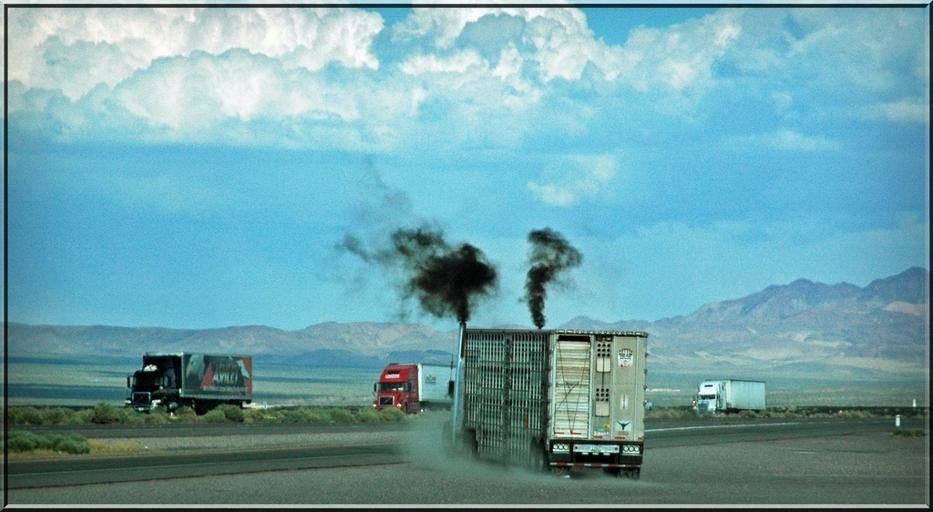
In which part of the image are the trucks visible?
[442,321,648,481]
[691,380,766,417]
[125,352,252,415]
[373,361,456,414]
